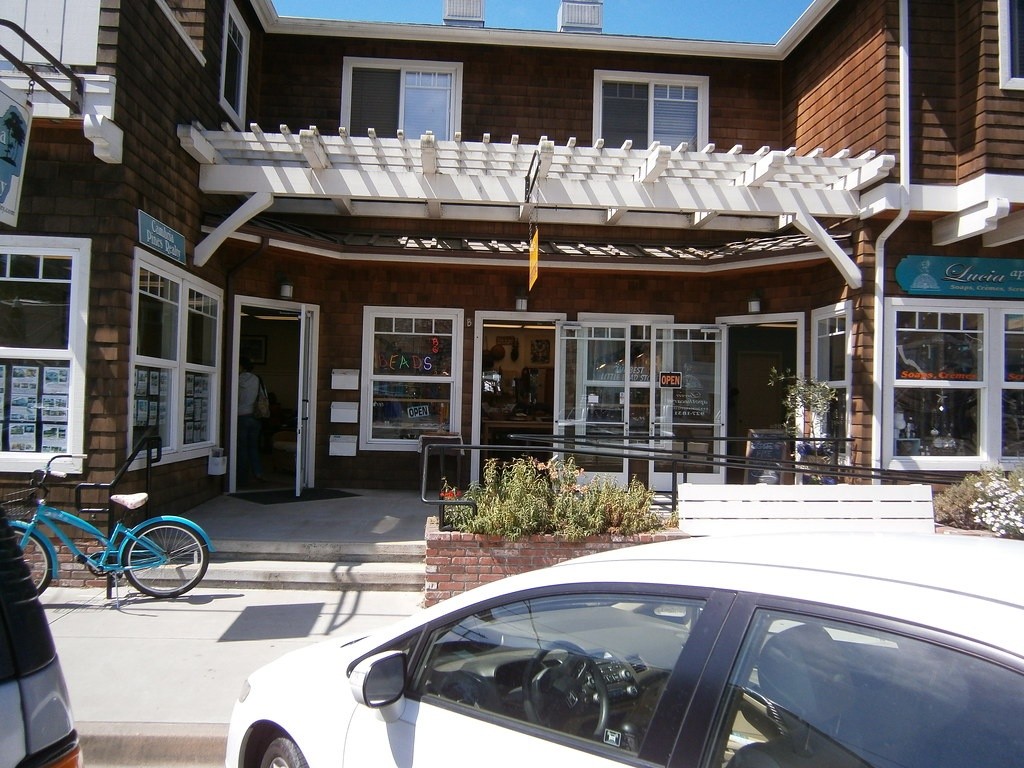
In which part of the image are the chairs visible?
[727,624,863,768]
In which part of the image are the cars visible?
[224,522,1024,768]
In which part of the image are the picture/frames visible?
[240,335,268,365]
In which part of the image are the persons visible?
[482,349,500,403]
[237,356,270,487]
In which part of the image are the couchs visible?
[271,430,297,473]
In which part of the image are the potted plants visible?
[426,453,691,611]
[767,367,842,474]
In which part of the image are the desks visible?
[482,403,553,479]
[418,435,463,494]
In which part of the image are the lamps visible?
[745,296,761,313]
[515,290,528,312]
[595,344,644,372]
[274,272,296,300]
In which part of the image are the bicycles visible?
[0,453,216,609]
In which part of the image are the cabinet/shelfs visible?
[372,398,450,431]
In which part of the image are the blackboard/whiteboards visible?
[672,388,711,419]
[742,429,789,485]
[592,362,650,394]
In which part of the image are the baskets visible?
[0,488,37,520]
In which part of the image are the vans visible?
[0,504,86,768]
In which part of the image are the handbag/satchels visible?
[256,374,270,418]
